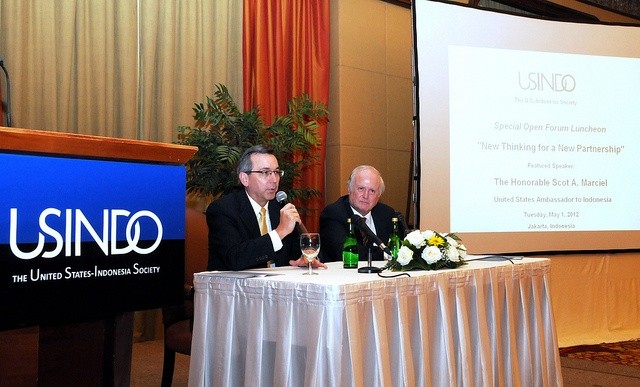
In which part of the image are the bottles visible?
[387,217,401,265]
[342,217,359,268]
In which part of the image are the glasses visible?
[244,170,284,178]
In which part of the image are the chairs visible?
[160,208,210,386]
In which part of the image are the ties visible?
[259,208,270,268]
[362,218,368,247]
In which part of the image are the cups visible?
[300,233,320,277]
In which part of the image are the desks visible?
[187,253,565,387]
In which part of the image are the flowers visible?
[395,228,467,270]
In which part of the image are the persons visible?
[205,145,328,270]
[319,165,404,263]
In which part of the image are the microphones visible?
[348,214,392,256]
[275,189,302,233]
